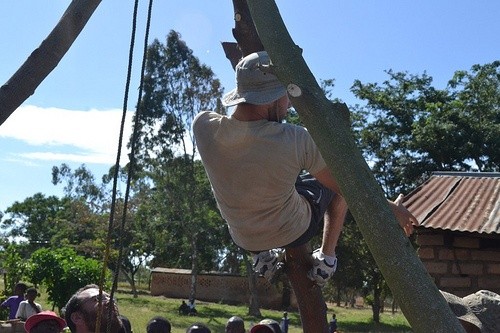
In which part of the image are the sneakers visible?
[251,247,287,281]
[307,248,339,287]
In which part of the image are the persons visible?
[15,288,43,322]
[438,288,500,333]
[119,300,337,333]
[64,283,126,333]
[24,310,66,333]
[0,283,29,320]
[191,50,420,289]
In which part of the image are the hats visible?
[25,311,67,331]
[438,290,500,332]
[251,319,282,333]
[220,51,288,108]
